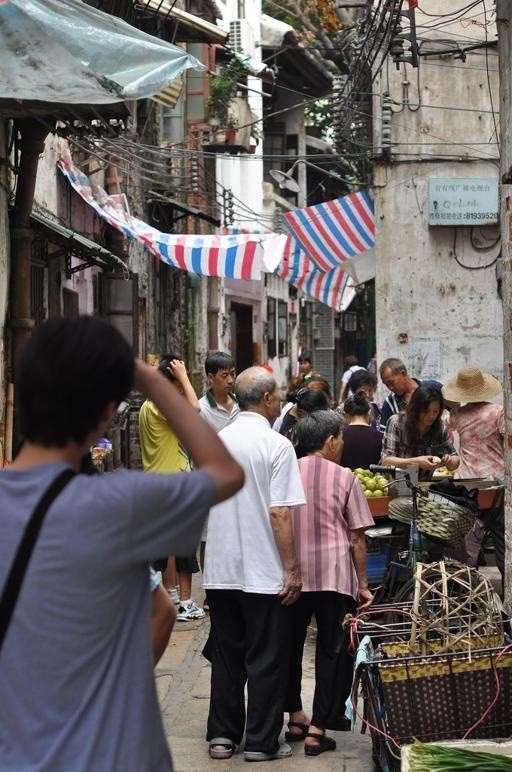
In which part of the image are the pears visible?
[344,467,389,497]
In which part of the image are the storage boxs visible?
[366,478,505,517]
[375,632,512,771]
[367,545,388,579]
[400,740,512,772]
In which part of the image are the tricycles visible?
[366,464,486,643]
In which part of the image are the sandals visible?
[285,718,312,741]
[209,737,236,758]
[303,732,337,756]
[243,742,294,762]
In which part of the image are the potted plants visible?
[201,47,264,153]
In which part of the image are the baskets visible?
[408,487,476,548]
[412,563,505,658]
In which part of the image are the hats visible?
[440,365,503,404]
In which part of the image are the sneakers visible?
[167,584,208,623]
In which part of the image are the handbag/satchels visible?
[373,634,512,745]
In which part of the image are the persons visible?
[284,408,377,758]
[137,351,505,623]
[1,314,247,772]
[7,437,178,672]
[200,364,308,762]
